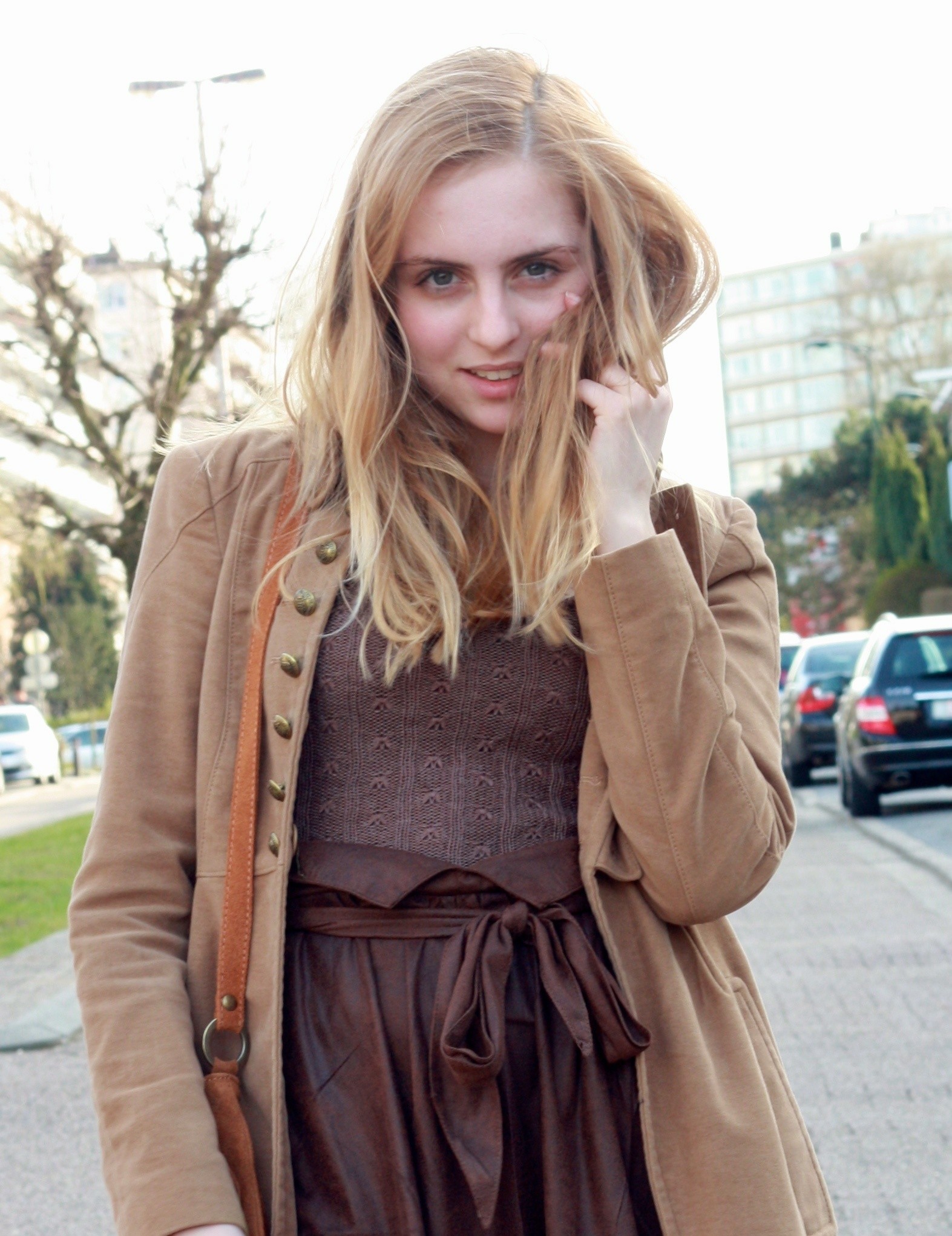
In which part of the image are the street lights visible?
[81,69,266,431]
[816,340,878,417]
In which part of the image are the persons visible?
[68,48,838,1236]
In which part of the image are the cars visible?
[54,720,106,770]
[777,630,948,789]
[0,704,63,785]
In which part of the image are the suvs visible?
[831,612,952,819]
